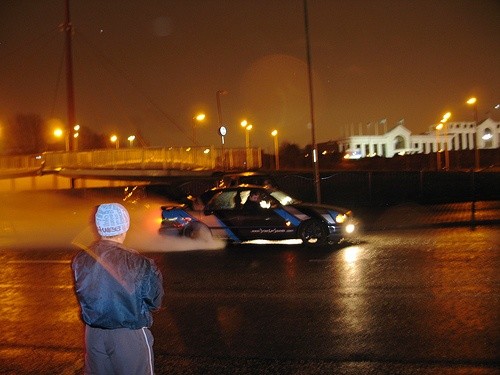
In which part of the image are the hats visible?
[95,202,130,237]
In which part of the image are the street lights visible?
[436,112,451,174]
[241,120,253,149]
[215,89,228,168]
[192,112,206,168]
[465,97,480,168]
[272,130,279,172]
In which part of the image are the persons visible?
[71,203,163,375]
[243,191,263,208]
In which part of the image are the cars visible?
[156,183,360,250]
